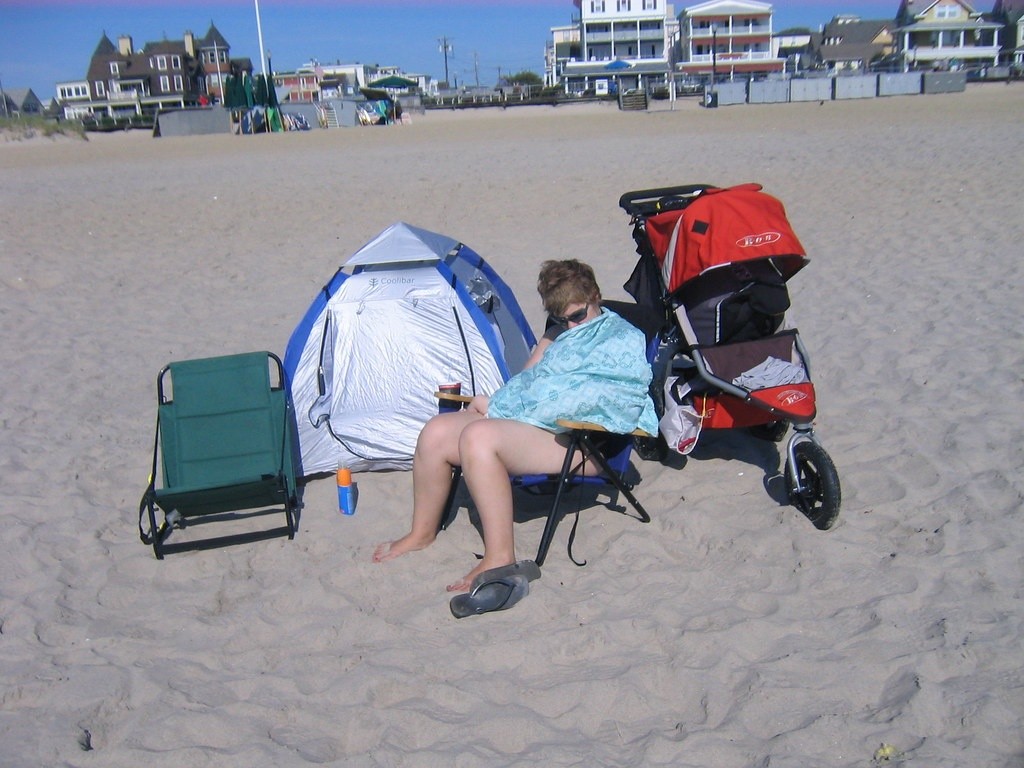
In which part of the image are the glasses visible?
[549,302,590,326]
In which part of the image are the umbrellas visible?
[368,76,418,105]
[225,74,284,136]
[604,60,631,78]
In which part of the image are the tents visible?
[281,221,539,479]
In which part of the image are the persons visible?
[396,106,402,119]
[198,95,208,107]
[373,260,653,593]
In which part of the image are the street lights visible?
[436,35,454,89]
[267,49,272,78]
[710,21,719,72]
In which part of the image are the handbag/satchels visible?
[687,274,790,345]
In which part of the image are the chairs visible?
[139,350,304,559]
[434,299,666,566]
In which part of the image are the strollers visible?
[598,184,843,530]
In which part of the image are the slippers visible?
[469,559,541,593]
[450,573,528,619]
